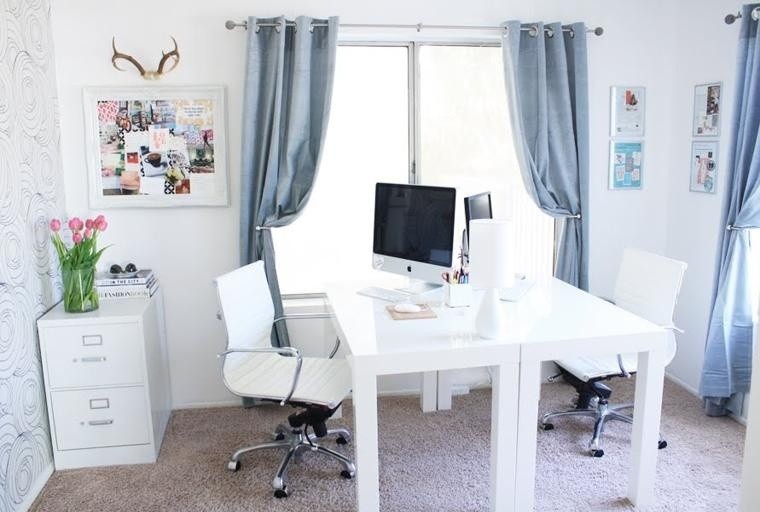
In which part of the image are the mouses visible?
[394,303,420,313]
[515,272,525,280]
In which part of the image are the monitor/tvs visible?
[372,182,457,285]
[464,191,493,252]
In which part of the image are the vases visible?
[62,270,99,312]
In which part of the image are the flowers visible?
[49,216,114,311]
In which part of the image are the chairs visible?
[539,249,688,457]
[213,260,351,499]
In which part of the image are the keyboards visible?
[500,279,536,302]
[357,287,411,303]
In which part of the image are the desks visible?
[323,275,677,512]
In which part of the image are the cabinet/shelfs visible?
[37,288,172,471]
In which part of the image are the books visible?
[94,269,162,299]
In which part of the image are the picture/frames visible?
[691,81,723,137]
[610,87,646,137]
[608,140,644,190]
[82,85,230,210]
[689,140,719,194]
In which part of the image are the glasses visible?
[110,263,136,273]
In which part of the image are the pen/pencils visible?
[442,266,468,284]
